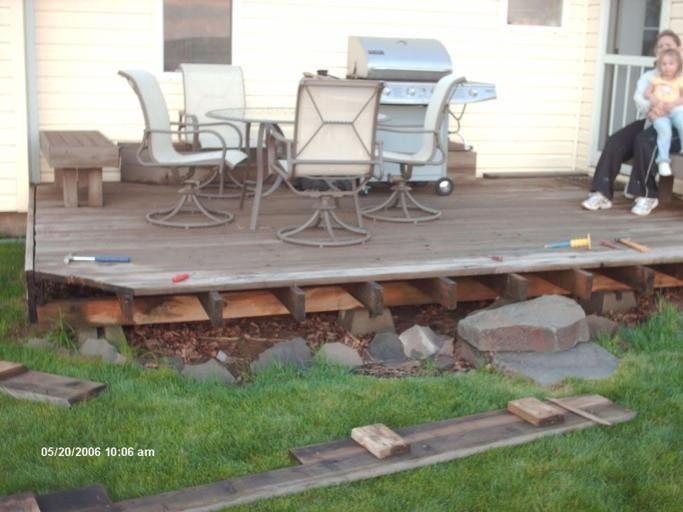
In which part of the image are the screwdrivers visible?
[172,271,196,283]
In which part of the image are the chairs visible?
[268,78,387,249]
[176,63,260,199]
[357,72,468,227]
[116,68,250,231]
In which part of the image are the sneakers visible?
[631,196,659,215]
[658,162,672,177]
[581,191,612,210]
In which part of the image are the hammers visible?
[63,253,130,264]
[615,235,649,253]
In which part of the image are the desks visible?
[204,108,295,234]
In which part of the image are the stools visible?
[622,148,682,209]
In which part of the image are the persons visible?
[579,30,683,217]
[642,49,682,177]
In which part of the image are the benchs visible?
[36,130,121,208]
